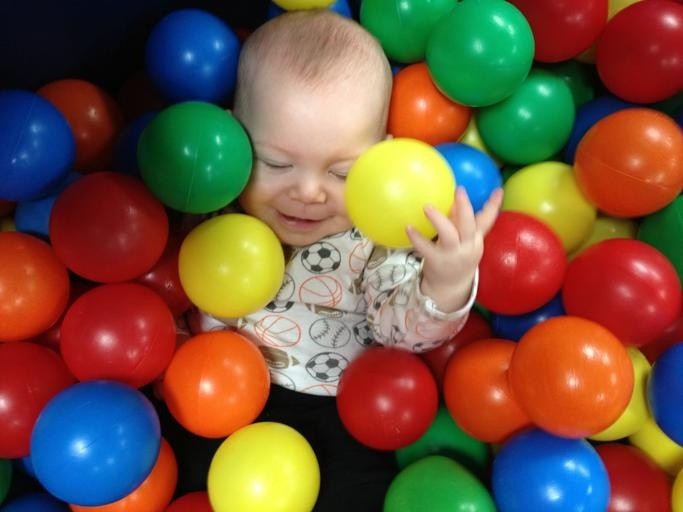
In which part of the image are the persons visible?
[139,10,505,512]
